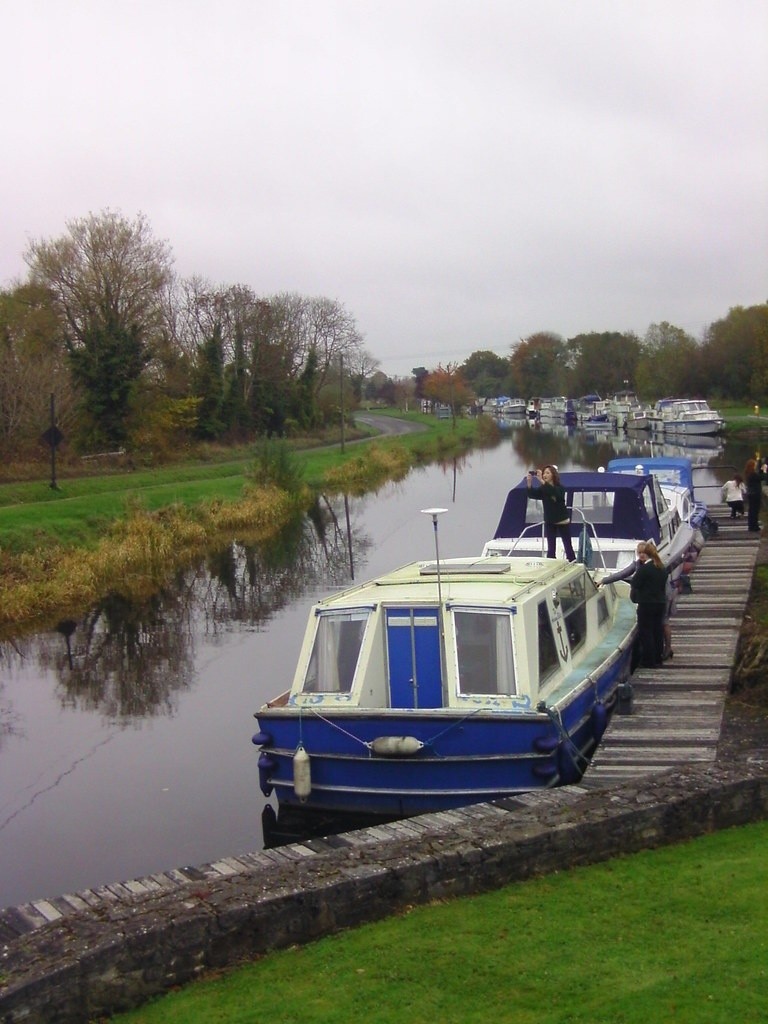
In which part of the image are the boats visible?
[252,507,642,816]
[482,379,724,434]
[480,465,695,590]
[607,457,709,529]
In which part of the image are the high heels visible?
[661,649,673,660]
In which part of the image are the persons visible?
[471,401,477,419]
[745,458,768,531]
[526,465,576,562]
[722,473,747,520]
[596,542,677,668]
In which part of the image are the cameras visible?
[529,470,537,476]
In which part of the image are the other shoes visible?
[748,527,761,531]
